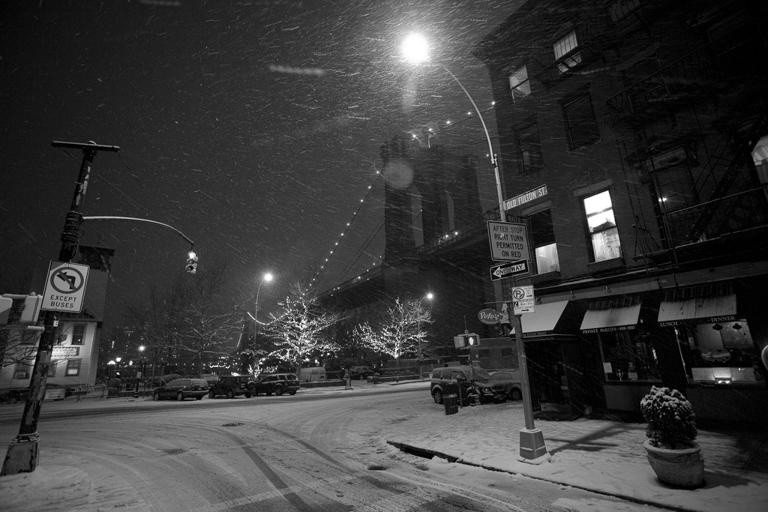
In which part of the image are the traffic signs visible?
[488,258,530,283]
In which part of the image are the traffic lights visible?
[185,251,200,274]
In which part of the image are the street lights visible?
[400,33,554,466]
[253,273,272,349]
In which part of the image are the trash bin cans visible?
[442,394,458,415]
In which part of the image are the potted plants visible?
[641,385,705,488]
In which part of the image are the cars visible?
[429,363,523,407]
[152,373,301,401]
[349,366,380,380]
